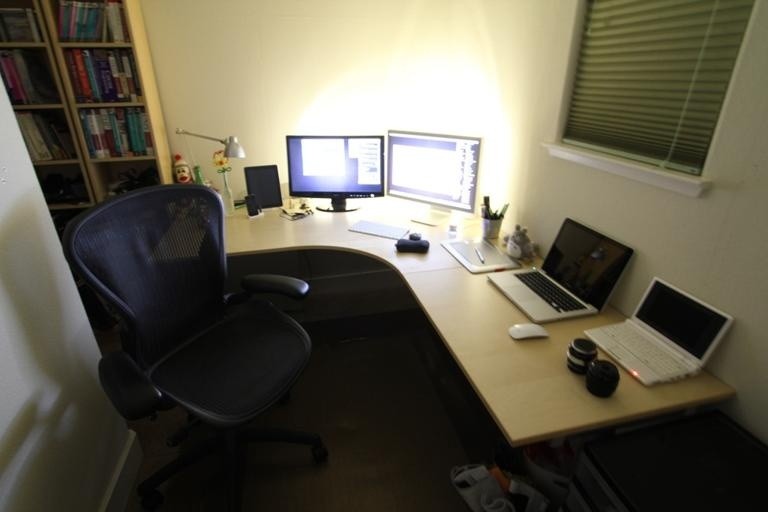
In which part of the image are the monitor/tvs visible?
[286,134,385,212]
[388,131,481,227]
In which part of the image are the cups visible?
[481,216,503,239]
[566,339,598,374]
[585,359,620,398]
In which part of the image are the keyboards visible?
[349,221,410,239]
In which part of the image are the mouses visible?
[509,323,549,340]
[411,233,421,240]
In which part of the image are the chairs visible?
[64,185,336,504]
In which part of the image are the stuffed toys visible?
[505,223,536,260]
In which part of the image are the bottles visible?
[192,166,205,185]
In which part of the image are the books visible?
[1,1,158,161]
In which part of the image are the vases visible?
[221,174,236,218]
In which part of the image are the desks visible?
[152,192,741,508]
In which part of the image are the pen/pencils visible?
[481,203,510,220]
[474,246,484,263]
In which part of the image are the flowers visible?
[210,150,233,198]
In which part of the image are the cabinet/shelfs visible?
[1,1,176,253]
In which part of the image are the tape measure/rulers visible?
[484,197,489,211]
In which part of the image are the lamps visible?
[173,126,246,170]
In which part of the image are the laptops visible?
[487,218,634,324]
[584,277,735,386]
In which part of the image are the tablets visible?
[245,164,283,209]
